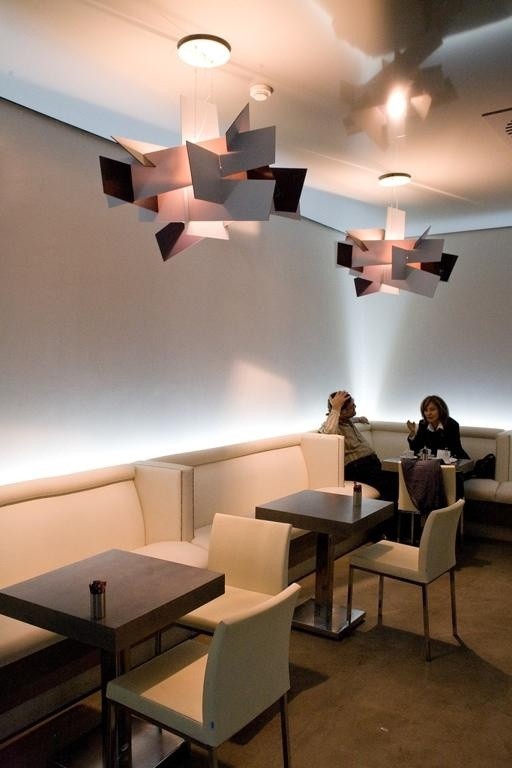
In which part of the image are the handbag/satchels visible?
[475,453,496,479]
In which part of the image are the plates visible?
[400,455,418,460]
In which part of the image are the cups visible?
[422,452,429,461]
[406,450,414,458]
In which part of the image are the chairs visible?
[346,500,464,660]
[106,582,303,768]
[172,513,291,637]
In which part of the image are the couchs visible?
[0,434,341,739]
[317,422,512,502]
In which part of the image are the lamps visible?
[335,172,458,297]
[99,34,307,263]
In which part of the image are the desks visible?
[255,489,393,641]
[381,455,477,541]
[0,548,224,768]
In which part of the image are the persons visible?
[318,391,399,542]
[404,395,470,499]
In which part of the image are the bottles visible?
[353,484,361,508]
[89,580,107,619]
